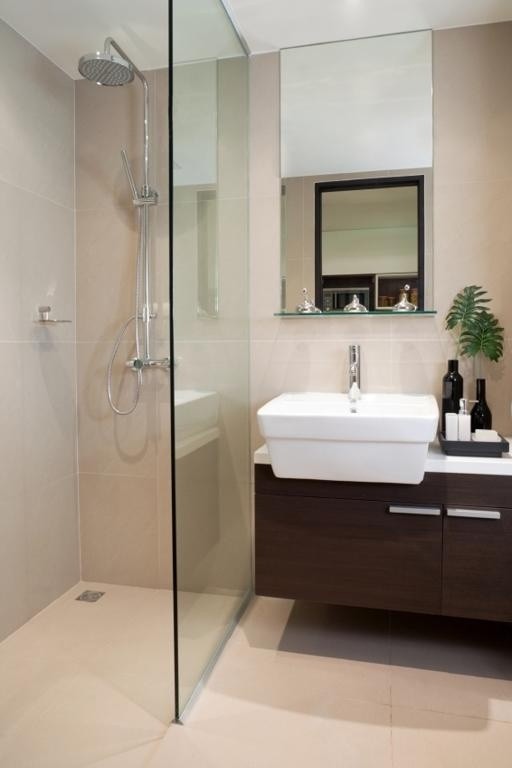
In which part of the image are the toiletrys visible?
[445,413,459,442]
[459,413,472,442]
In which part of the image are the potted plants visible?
[460,311,504,432]
[441,286,492,432]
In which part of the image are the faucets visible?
[349,344,360,397]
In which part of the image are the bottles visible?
[441,359,464,435]
[470,378,493,433]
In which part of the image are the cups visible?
[445,412,471,442]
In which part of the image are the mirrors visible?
[274,29,437,316]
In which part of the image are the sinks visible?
[259,393,439,486]
[175,389,220,456]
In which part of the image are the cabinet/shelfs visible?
[255,464,512,624]
